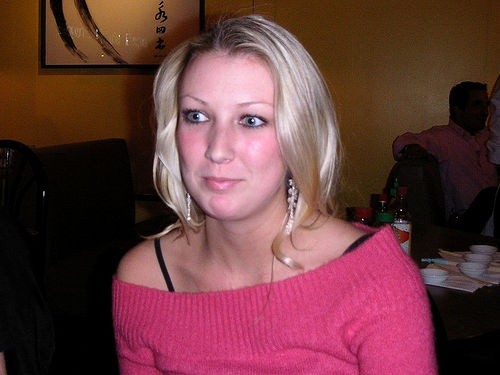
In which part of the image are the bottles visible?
[389,165,401,198]
[377,194,392,225]
[393,188,411,257]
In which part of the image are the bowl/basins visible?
[456,262,489,276]
[422,269,448,283]
[470,245,497,255]
[464,253,492,263]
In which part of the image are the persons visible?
[112,15,437,375]
[392,82,500,236]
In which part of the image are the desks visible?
[410,221,500,355]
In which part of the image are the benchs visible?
[22,141,137,325]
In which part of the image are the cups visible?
[356,208,372,226]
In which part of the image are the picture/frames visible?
[37,0,206,75]
